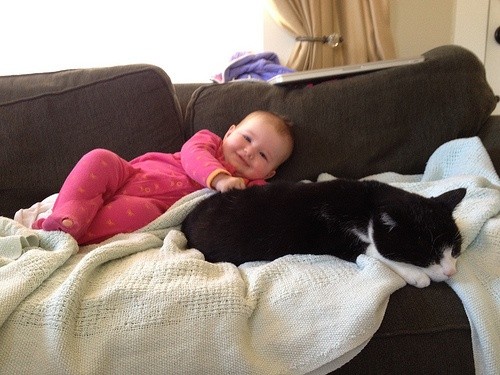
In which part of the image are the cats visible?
[181,178,467,288]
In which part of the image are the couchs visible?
[0,43,500,375]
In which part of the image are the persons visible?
[32,109,295,246]
